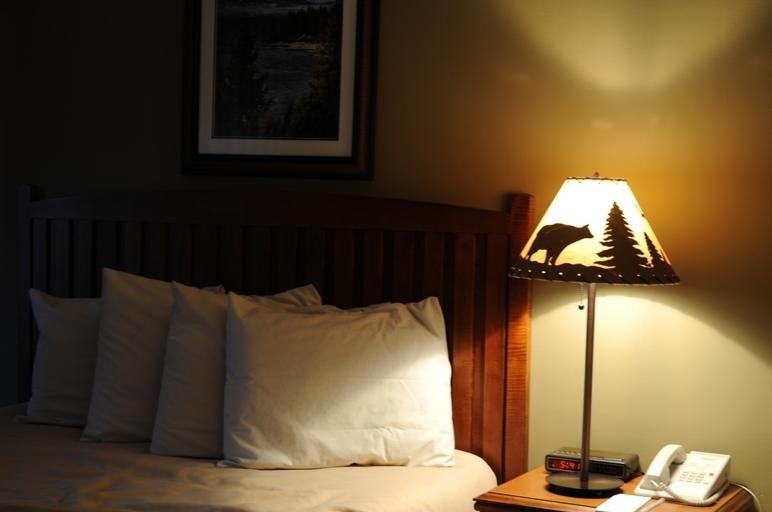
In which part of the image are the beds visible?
[0,189,536,511]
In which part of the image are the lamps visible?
[507,171,683,496]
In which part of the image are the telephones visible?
[633,442,733,508]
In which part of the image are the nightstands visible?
[474,462,754,512]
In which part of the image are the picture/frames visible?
[178,0,375,182]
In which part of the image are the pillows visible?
[149,281,228,460]
[15,287,101,427]
[78,267,323,442]
[216,290,455,470]
